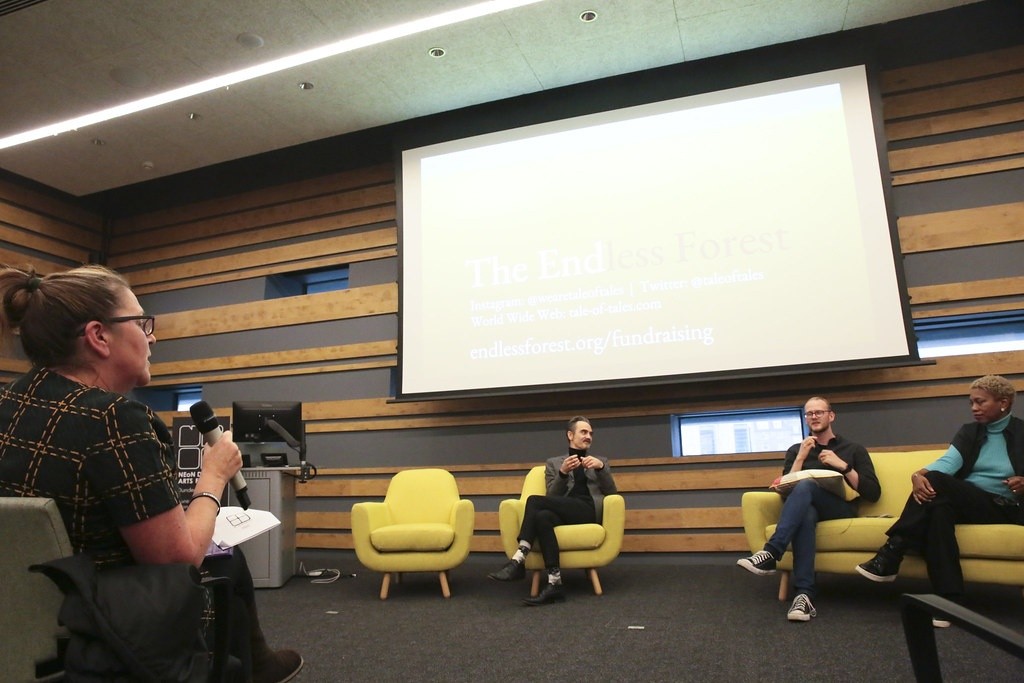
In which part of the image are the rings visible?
[913,474,917,476]
[1013,490,1017,493]
[821,457,824,460]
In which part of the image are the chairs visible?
[351,468,475,598]
[499,466,626,596]
[0,496,241,683]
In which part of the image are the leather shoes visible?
[523,583,566,606]
[487,562,527,583]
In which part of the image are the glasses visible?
[804,409,832,419]
[74,313,155,338]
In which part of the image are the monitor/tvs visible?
[232,401,303,444]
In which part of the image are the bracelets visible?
[842,464,852,474]
[188,493,220,516]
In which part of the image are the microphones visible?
[191,401,251,511]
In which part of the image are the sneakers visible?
[786,593,817,620]
[736,549,778,575]
[932,612,951,628]
[856,545,904,582]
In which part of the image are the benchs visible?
[742,449,1023,601]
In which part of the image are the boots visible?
[244,628,305,683]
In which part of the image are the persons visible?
[487,416,616,606]
[1,262,305,683]
[856,376,1024,628]
[738,397,882,621]
